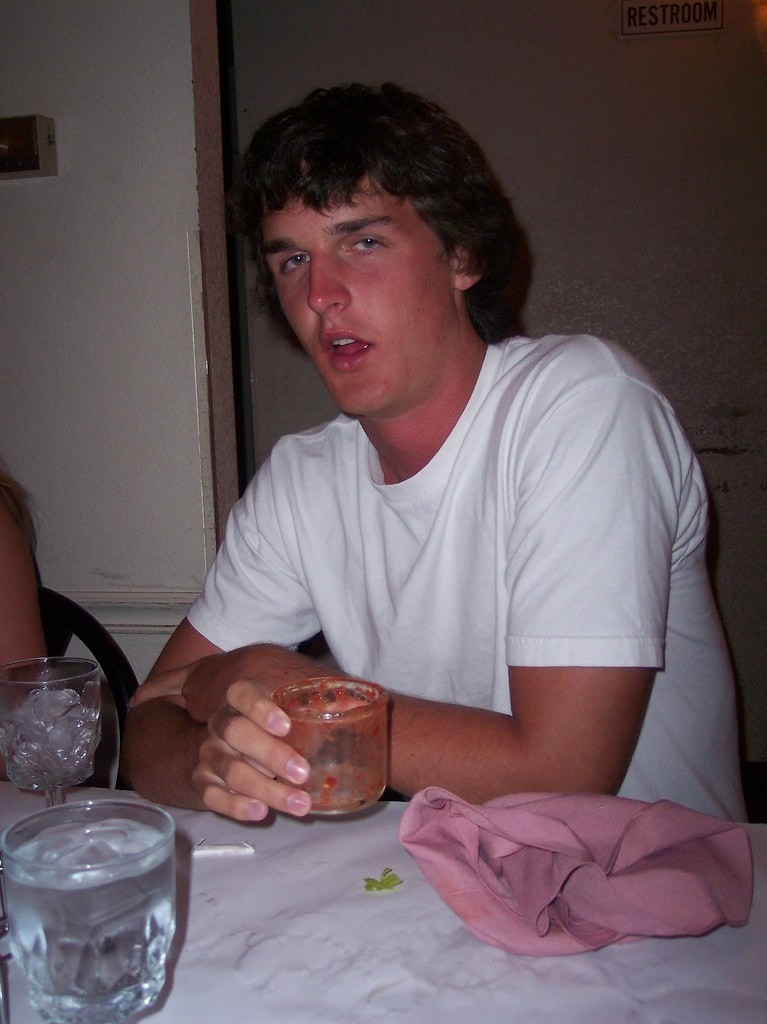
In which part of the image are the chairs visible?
[36,583,141,790]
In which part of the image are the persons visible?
[119,80,747,824]
[0,455,54,782]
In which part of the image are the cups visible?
[1,798,177,1024]
[271,677,388,816]
[0,657,101,809]
